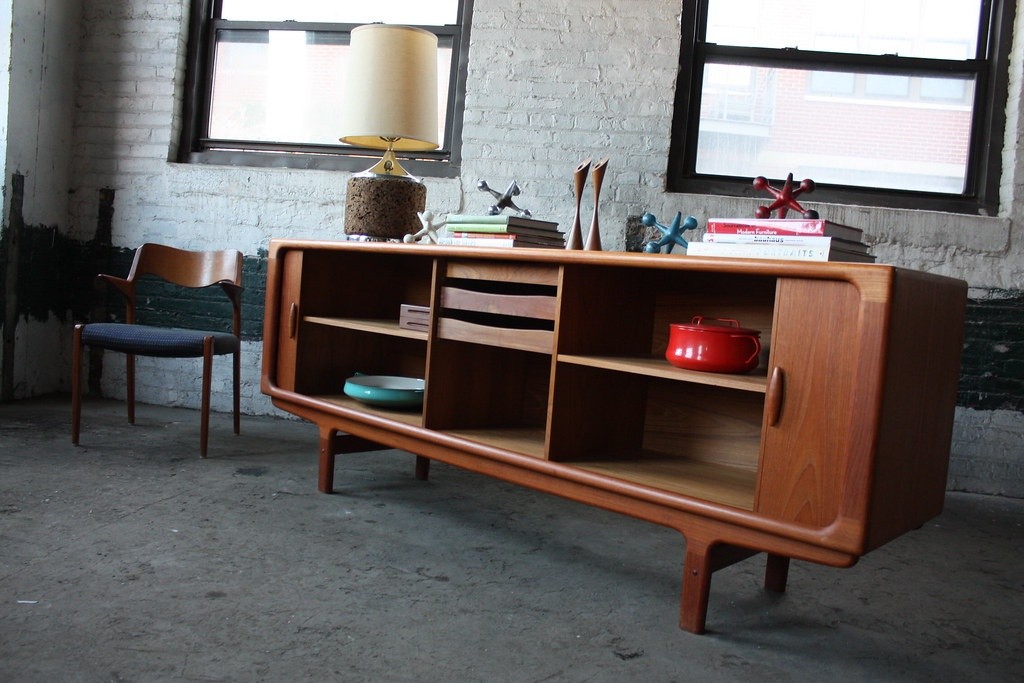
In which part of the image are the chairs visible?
[72,242,243,459]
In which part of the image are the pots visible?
[665,316,763,372]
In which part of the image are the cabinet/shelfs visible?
[260,237,969,635]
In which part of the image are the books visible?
[437,214,565,250]
[687,218,879,263]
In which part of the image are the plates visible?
[343,376,427,408]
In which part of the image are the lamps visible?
[338,23,439,243]
